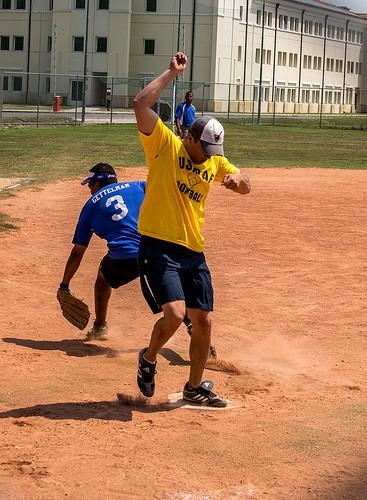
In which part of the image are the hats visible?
[189,115,225,156]
[81,170,116,185]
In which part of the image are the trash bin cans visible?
[53,96,61,111]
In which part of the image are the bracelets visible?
[60,283,69,288]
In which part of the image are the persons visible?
[133,52,251,408]
[175,91,196,139]
[56,163,217,358]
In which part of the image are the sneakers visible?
[187,323,217,358]
[86,320,109,339]
[136,347,157,397]
[183,380,227,407]
